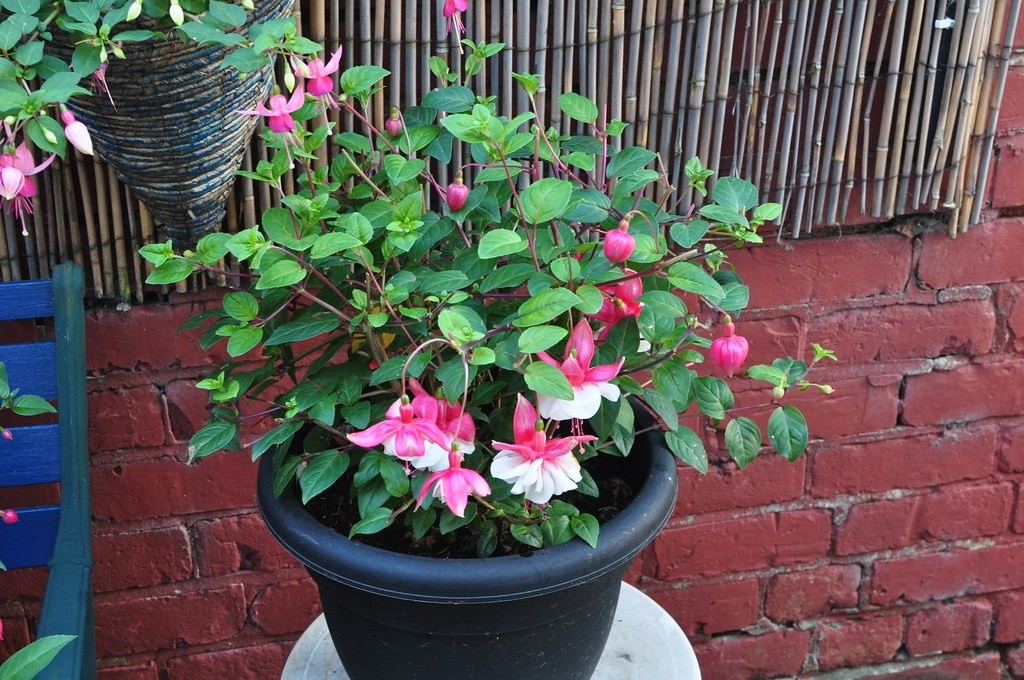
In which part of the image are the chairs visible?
[0,259,95,680]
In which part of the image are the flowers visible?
[134,1,838,558]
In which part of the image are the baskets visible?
[27,0,295,254]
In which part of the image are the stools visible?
[281,580,702,680]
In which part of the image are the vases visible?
[255,428,680,680]
